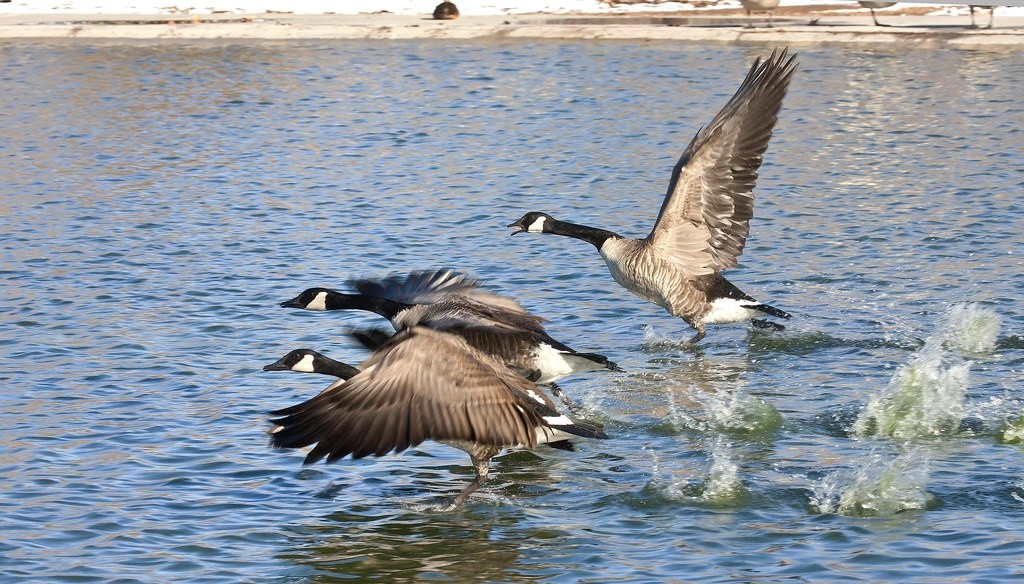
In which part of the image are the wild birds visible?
[280,265,628,409]
[506,43,801,345]
[259,326,612,515]
